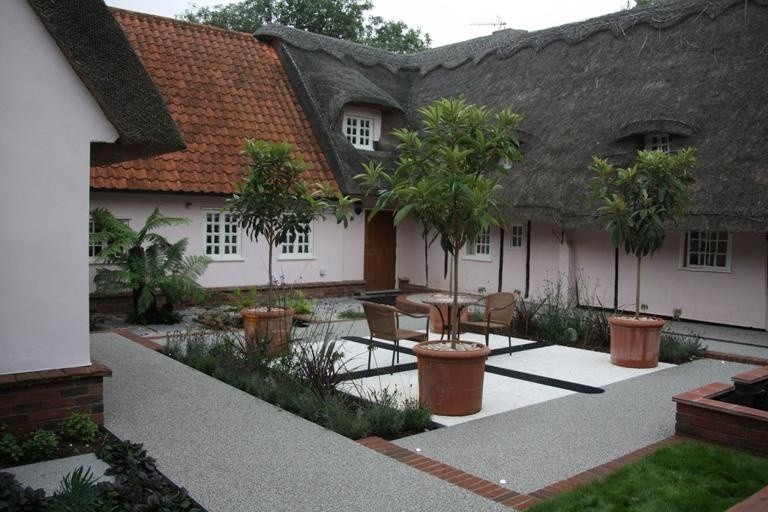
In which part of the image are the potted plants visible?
[355,94,526,417]
[226,137,361,358]
[585,147,699,370]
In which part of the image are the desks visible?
[419,295,479,343]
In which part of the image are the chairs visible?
[457,292,517,360]
[361,301,430,374]
